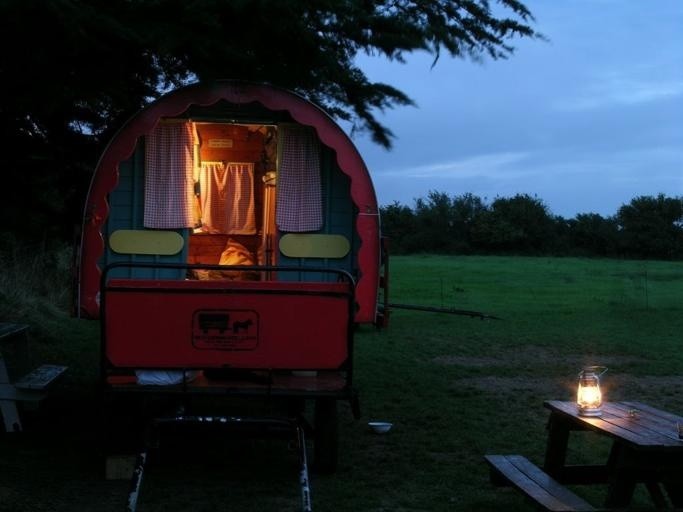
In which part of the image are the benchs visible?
[485,453,597,511]
[15,362,67,401]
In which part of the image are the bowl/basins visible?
[368,421,392,433]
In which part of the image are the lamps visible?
[574,364,609,417]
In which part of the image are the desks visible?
[0,319,69,433]
[492,399,683,511]
